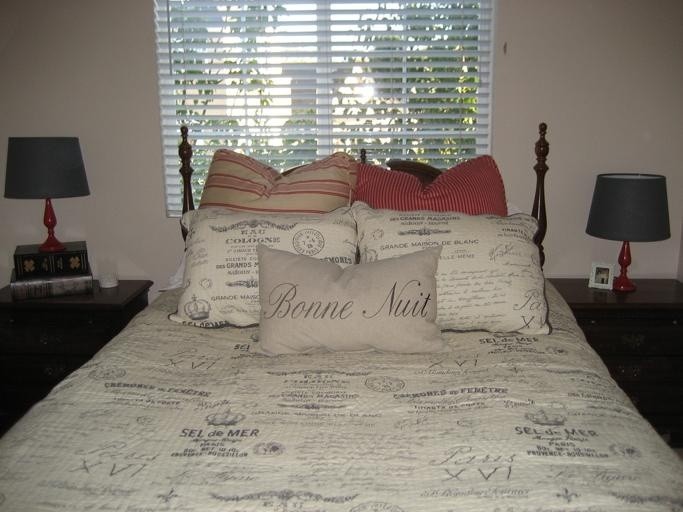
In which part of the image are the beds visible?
[1,121,682,511]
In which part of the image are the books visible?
[9,240,101,302]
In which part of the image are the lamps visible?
[586,174,672,290]
[2,137,90,251]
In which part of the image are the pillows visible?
[197,148,357,213]
[353,153,507,218]
[166,205,358,331]
[388,159,441,186]
[351,199,553,336]
[247,243,453,357]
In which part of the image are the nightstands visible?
[0,279,155,433]
[549,277,682,447]
